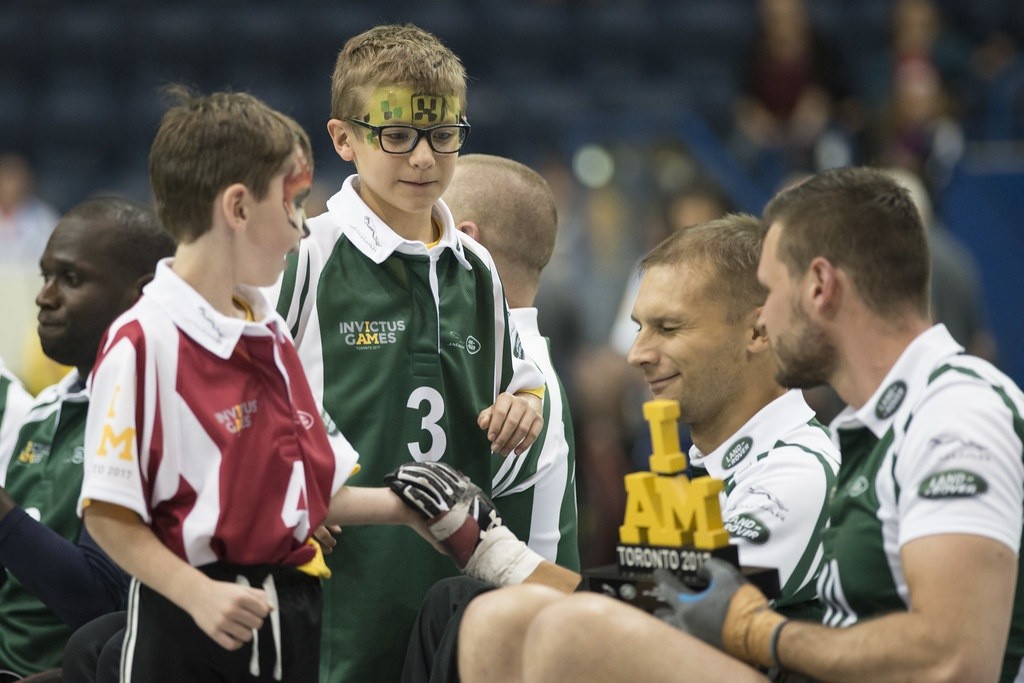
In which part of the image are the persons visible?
[383,215,841,683]
[458,168,1024,683]
[0,197,178,683]
[76,92,451,683]
[439,154,578,573]
[259,25,547,683]
[577,0,995,541]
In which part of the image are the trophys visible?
[587,400,782,617]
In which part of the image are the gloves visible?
[653,559,791,683]
[384,461,545,588]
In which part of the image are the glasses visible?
[342,117,471,154]
